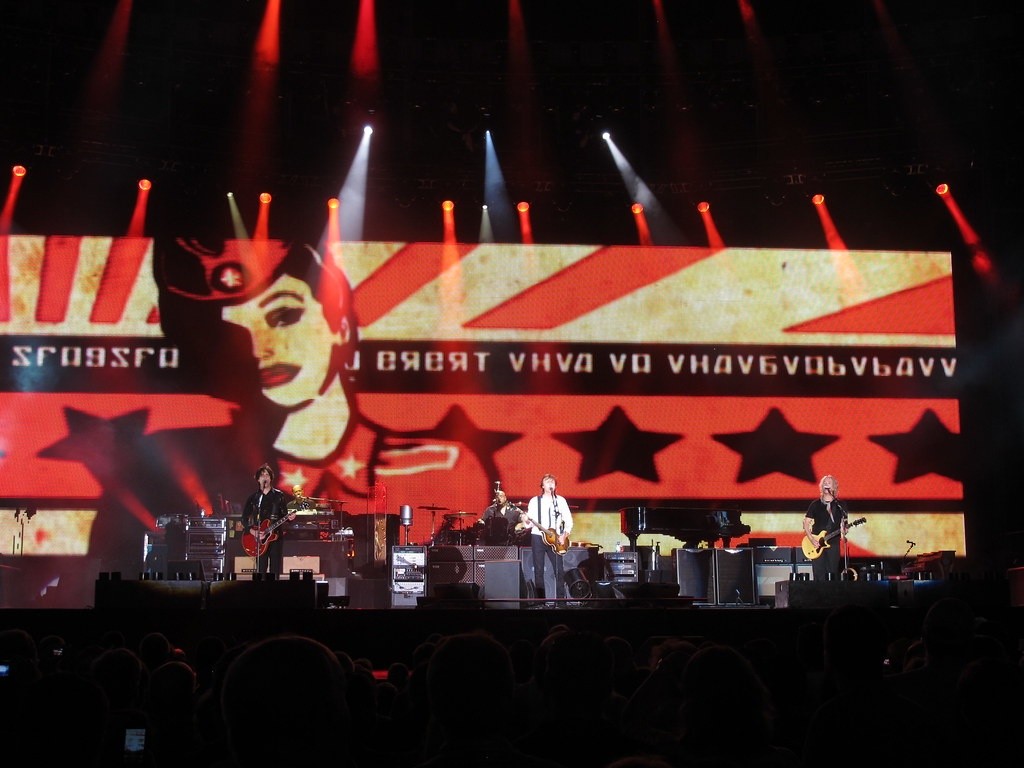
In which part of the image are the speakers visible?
[98,514,947,609]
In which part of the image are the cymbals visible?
[445,511,478,518]
[417,505,450,510]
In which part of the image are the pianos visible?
[618,507,751,551]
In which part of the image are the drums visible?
[485,517,508,541]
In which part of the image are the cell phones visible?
[123,729,146,764]
[0,664,9,677]
[53,647,64,656]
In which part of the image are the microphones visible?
[550,487,553,497]
[824,487,830,489]
[263,481,266,488]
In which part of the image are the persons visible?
[286,485,317,513]
[240,466,289,583]
[519,476,573,608]
[804,474,846,579]
[1,592,1024,768]
[616,542,622,552]
[481,490,524,532]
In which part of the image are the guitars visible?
[242,510,298,557]
[528,516,572,554]
[802,516,868,558]
